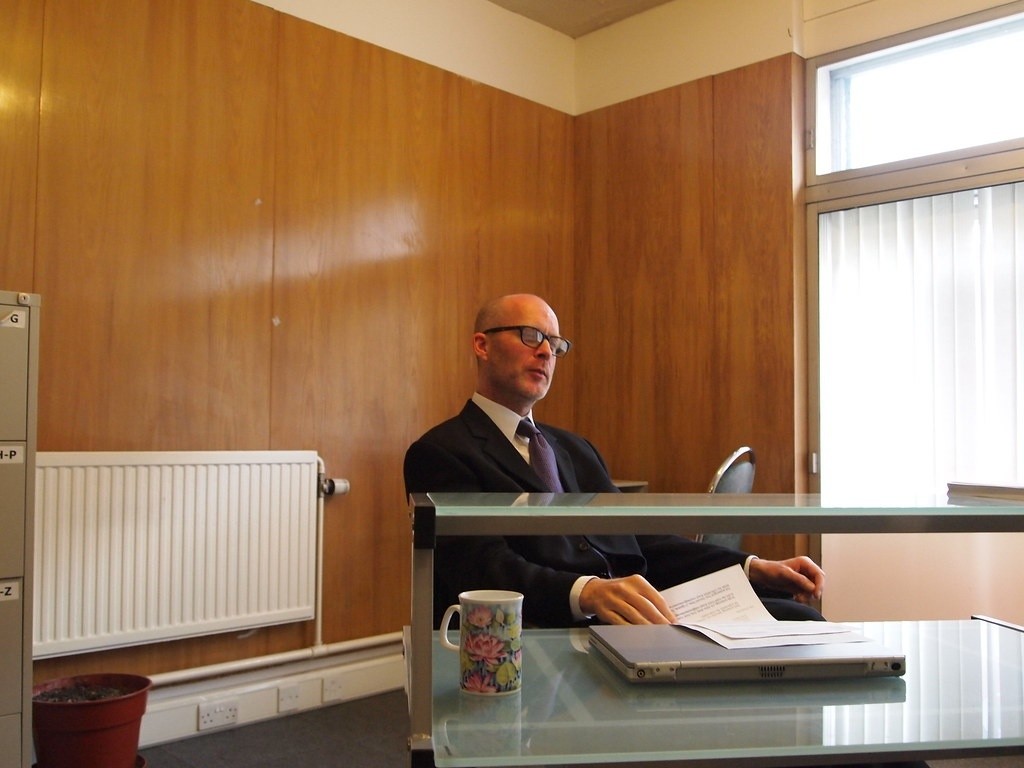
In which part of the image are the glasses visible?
[483,325,571,359]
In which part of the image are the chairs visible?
[696,446,757,548]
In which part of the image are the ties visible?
[516,417,564,494]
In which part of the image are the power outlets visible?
[323,674,345,701]
[198,698,240,731]
[278,684,300,713]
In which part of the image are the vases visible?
[31,673,154,768]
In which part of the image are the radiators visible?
[31,451,349,662]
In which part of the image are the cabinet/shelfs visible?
[0,290,41,768]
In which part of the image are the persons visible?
[402,293,828,630]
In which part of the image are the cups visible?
[438,590,524,696]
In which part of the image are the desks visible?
[400,493,1024,768]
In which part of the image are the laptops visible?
[587,625,906,682]
